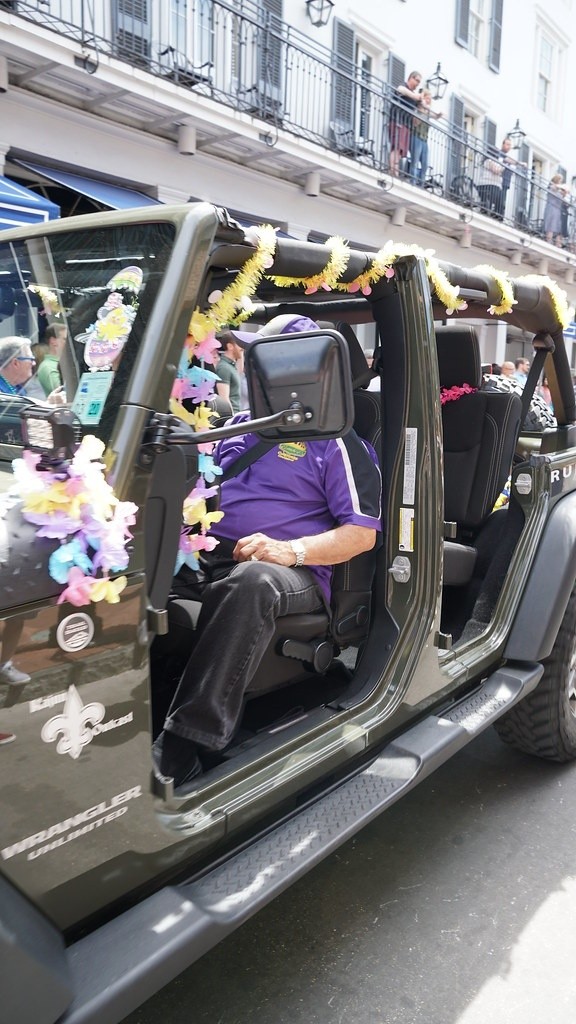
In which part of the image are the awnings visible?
[0,175,60,230]
[486,322,576,339]
[12,158,165,212]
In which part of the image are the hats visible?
[230,314,321,351]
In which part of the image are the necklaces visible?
[0,373,16,395]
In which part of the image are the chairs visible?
[404,157,445,197]
[236,84,292,127]
[153,45,214,99]
[450,175,505,219]
[328,121,376,169]
[169,317,382,700]
[517,210,544,239]
[437,317,525,588]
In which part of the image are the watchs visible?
[289,540,305,567]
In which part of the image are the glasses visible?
[15,356,36,364]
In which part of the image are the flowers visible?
[14,431,140,603]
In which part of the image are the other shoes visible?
[151,744,203,790]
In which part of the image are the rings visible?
[250,554,259,562]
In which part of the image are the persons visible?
[477,138,527,221]
[543,175,576,246]
[183,332,249,415]
[0,287,137,406]
[389,72,445,187]
[155,314,384,787]
[493,358,552,410]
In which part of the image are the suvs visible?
[0,205,576,1024]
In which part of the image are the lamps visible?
[537,260,548,276]
[425,62,449,100]
[564,269,574,285]
[459,229,472,248]
[306,0,334,27]
[177,124,197,155]
[510,251,522,265]
[506,119,527,150]
[304,172,320,196]
[390,207,406,226]
